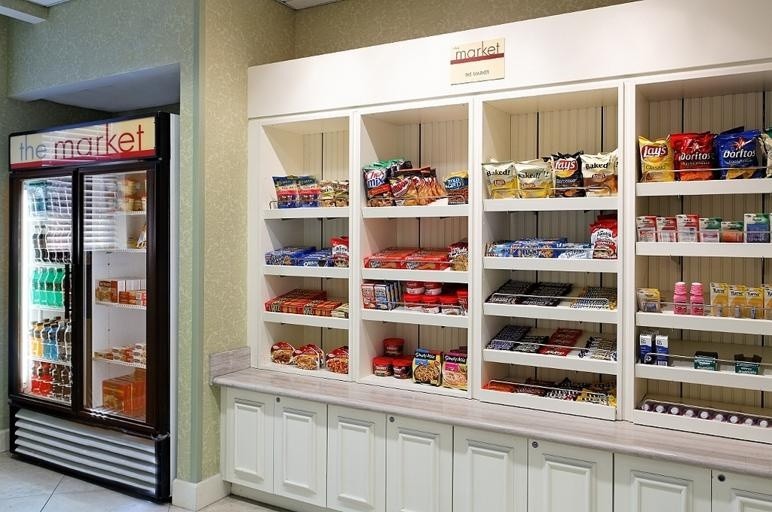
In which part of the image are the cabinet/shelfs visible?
[356,96,475,399]
[220,382,325,510]
[613,449,771,511]
[474,78,622,424]
[327,402,452,511]
[623,59,772,446]
[249,110,354,382]
[453,422,613,511]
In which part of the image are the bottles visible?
[27,181,73,404]
[673,282,689,316]
[690,282,705,316]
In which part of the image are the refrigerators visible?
[6,109,182,504]
